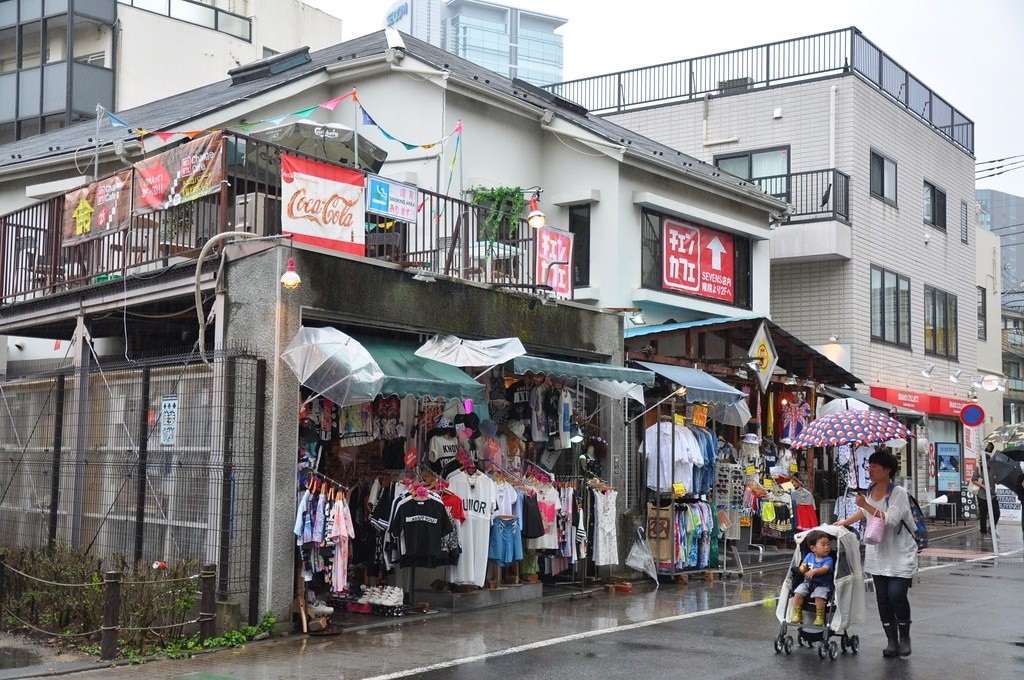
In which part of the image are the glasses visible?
[714,464,744,509]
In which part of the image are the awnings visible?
[312,330,486,406]
[633,360,749,405]
[513,354,656,388]
[823,385,926,427]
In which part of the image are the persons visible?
[971,453,1001,539]
[790,530,833,626]
[833,450,919,658]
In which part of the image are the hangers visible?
[295,371,874,528]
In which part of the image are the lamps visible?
[878,362,1006,398]
[527,195,546,229]
[628,311,646,325]
[280,237,301,290]
[538,289,559,307]
[411,268,437,283]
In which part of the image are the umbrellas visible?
[707,398,752,427]
[983,422,1024,498]
[818,397,870,415]
[788,399,916,494]
[247,119,388,179]
[414,334,526,367]
[279,325,386,407]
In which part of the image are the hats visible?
[437,418,454,428]
[743,433,758,444]
[508,420,527,441]
[781,438,791,445]
[482,419,500,439]
[461,412,482,439]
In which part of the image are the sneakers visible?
[309,599,333,614]
[330,584,404,606]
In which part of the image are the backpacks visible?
[867,482,929,551]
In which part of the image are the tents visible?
[195,138,282,203]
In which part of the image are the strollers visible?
[773,525,861,660]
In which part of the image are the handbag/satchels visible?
[863,509,885,545]
[969,465,982,495]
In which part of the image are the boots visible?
[881,620,899,656]
[814,610,825,625]
[897,618,912,656]
[792,606,801,623]
